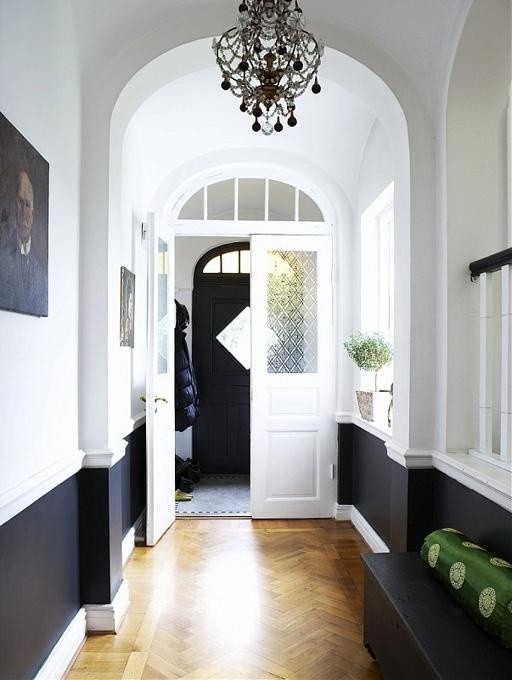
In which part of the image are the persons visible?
[1,165,48,312]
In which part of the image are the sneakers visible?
[176,454,200,501]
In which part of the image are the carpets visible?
[171,472,252,519]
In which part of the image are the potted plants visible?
[340,329,393,422]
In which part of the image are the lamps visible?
[209,1,326,137]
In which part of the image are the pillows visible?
[421,526,512,647]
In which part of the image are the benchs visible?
[359,550,512,679]
